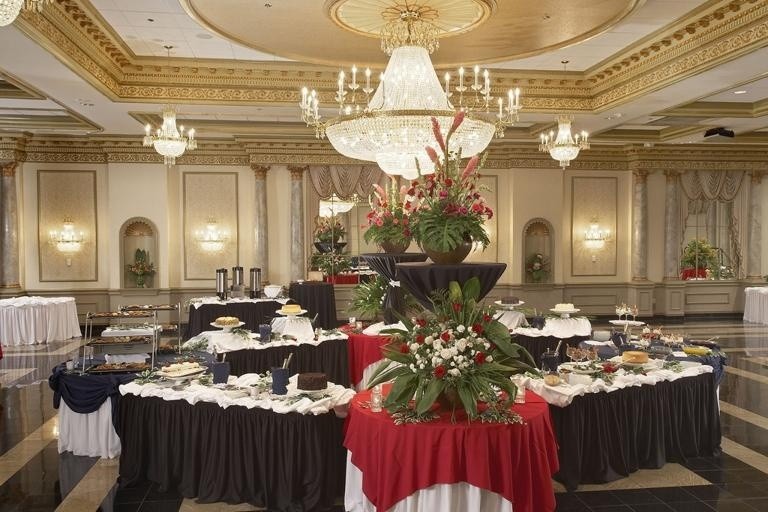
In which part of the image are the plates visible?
[155,296,719,397]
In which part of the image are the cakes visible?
[555,303,574,311]
[215,317,239,325]
[500,297,520,304]
[298,372,327,390]
[161,358,200,375]
[622,351,649,363]
[282,304,301,313]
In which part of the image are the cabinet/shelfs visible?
[117,303,182,355]
[82,310,158,373]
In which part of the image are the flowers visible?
[405,115,493,252]
[344,273,390,317]
[313,212,347,241]
[360,172,416,241]
[316,251,346,274]
[358,278,545,419]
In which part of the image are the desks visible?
[339,323,390,382]
[342,380,560,512]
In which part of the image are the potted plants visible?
[684,237,725,269]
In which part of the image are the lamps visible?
[192,215,230,255]
[540,60,591,169]
[300,7,524,179]
[144,42,197,167]
[582,221,610,262]
[46,211,88,267]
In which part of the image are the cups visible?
[66,361,74,370]
[338,263,379,274]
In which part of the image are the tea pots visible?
[717,266,740,280]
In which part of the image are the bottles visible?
[231,266,243,287]
[214,268,228,301]
[250,267,261,300]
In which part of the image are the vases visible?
[377,235,412,253]
[314,243,345,252]
[423,241,471,263]
